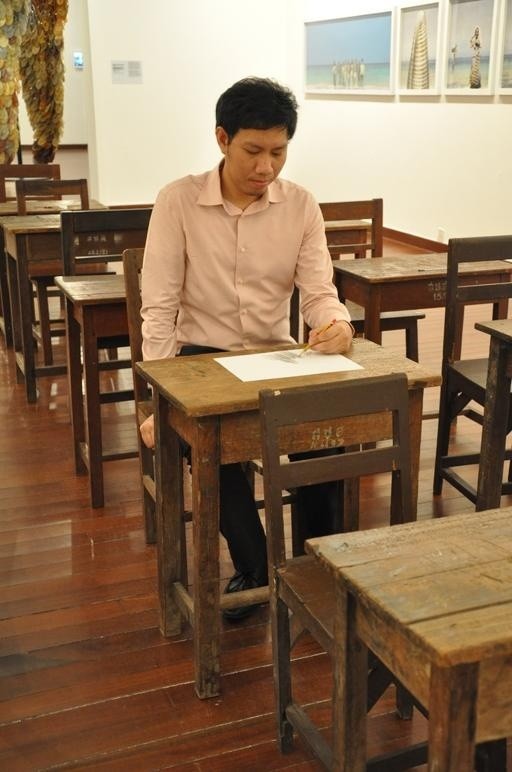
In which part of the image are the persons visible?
[137,76,356,622]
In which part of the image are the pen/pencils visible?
[297,320,336,357]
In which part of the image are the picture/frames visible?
[443,0,501,97]
[495,0,512,96]
[394,1,442,97]
[302,6,396,97]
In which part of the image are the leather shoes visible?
[220,549,268,622]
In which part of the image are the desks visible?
[305,505,510,771]
[136,341,444,696]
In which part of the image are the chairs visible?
[121,243,294,586]
[258,374,434,770]
[1,158,509,511]
